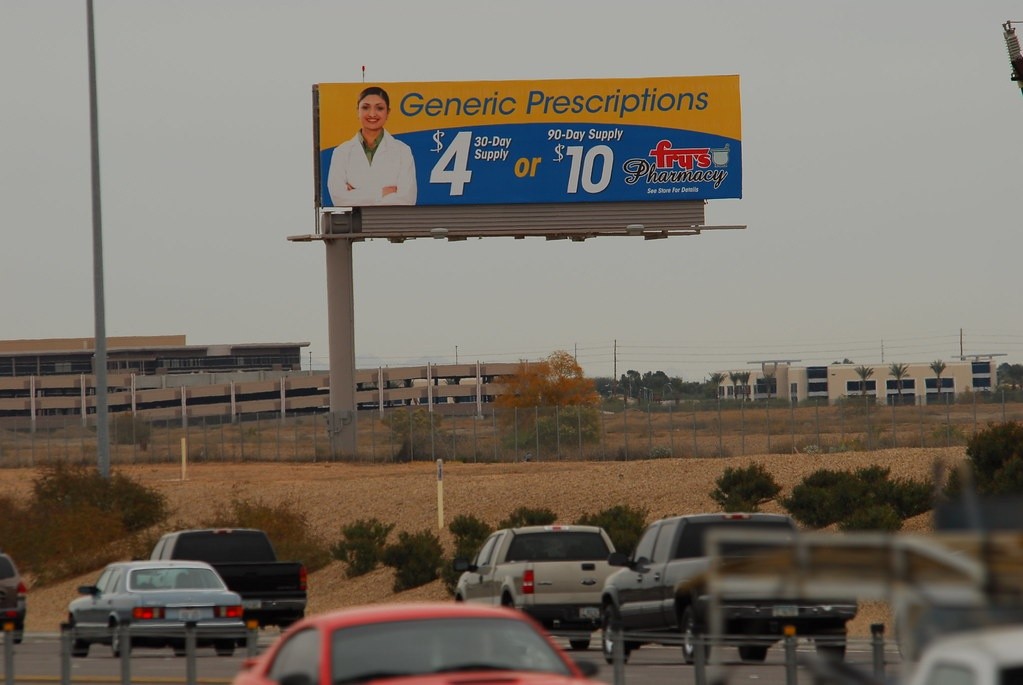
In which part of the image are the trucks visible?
[148,528,308,649]
[597,512,860,665]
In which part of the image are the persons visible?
[327,85,418,207]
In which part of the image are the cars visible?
[62,560,244,658]
[0,552,28,644]
[230,601,607,685]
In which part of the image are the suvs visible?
[450,525,627,651]
